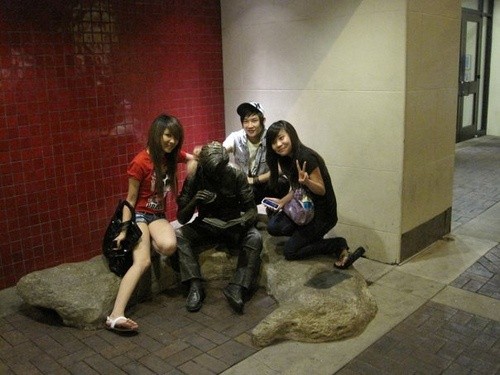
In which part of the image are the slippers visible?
[333,245,366,269]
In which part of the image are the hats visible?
[237,101,266,121]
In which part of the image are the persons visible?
[176,140,263,314]
[265,120,366,270]
[219,102,288,221]
[105,113,198,334]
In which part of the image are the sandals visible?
[105,315,138,332]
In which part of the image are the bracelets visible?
[253,176,260,186]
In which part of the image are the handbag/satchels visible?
[282,178,314,226]
[101,199,143,277]
